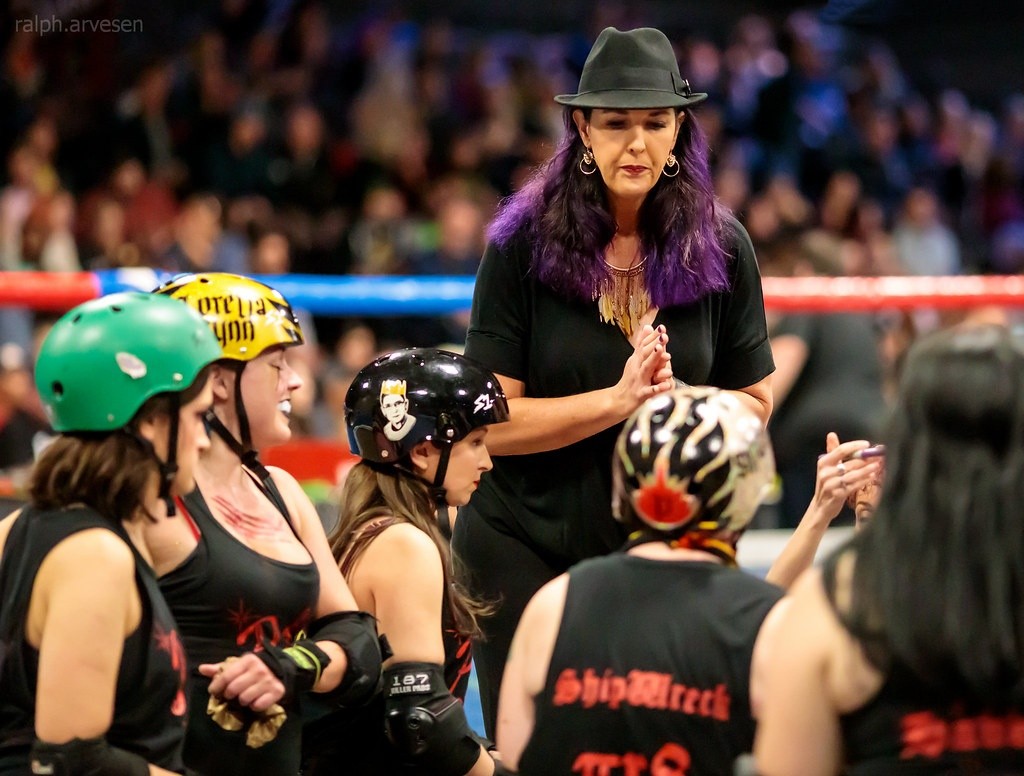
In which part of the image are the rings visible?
[839,476,846,488]
[837,464,845,475]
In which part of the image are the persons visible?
[498,387,784,776]
[765,432,883,592]
[1,2,1024,499]
[452,28,776,749]
[751,322,1024,774]
[326,346,510,776]
[769,231,886,528]
[141,271,382,776]
[2,291,223,776]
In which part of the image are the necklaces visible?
[593,252,650,337]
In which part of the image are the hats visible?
[554,26,708,110]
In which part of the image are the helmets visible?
[611,387,776,531]
[152,273,305,364]
[34,292,222,434]
[345,347,510,462]
